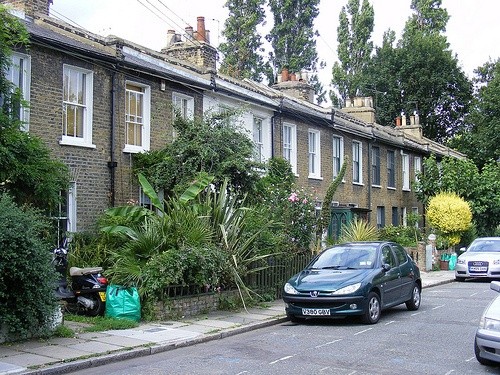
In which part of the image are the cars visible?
[474,280,500,368]
[282,241,422,324]
[455,237,500,282]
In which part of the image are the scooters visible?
[53,242,107,318]
